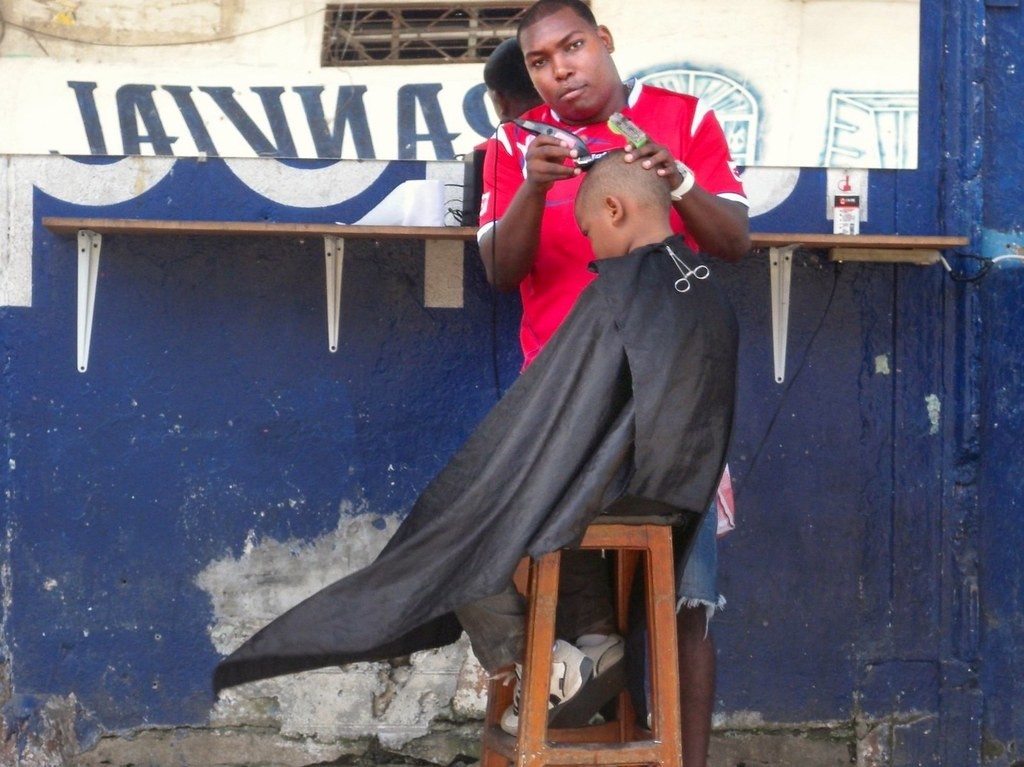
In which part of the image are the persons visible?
[471,2,750,765]
[410,146,739,739]
[462,37,548,304]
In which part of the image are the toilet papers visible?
[400,177,447,227]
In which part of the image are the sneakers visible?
[561,632,638,727]
[500,640,593,737]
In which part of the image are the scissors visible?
[666,246,710,293]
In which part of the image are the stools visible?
[480,514,684,767]
